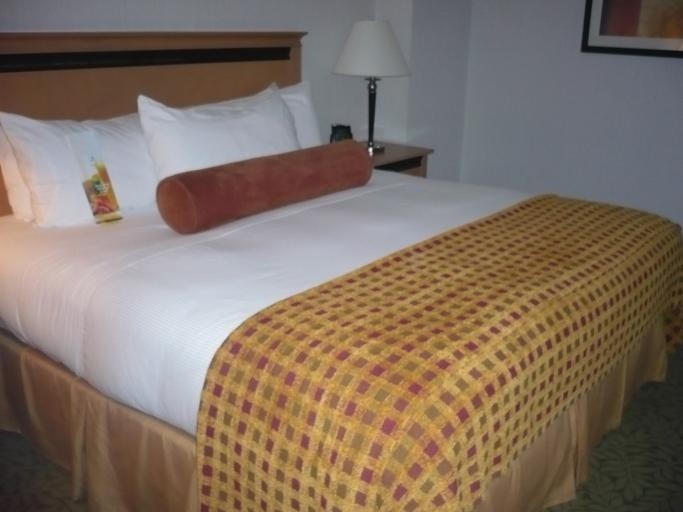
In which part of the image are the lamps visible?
[330,19,411,152]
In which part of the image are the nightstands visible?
[358,140,434,178]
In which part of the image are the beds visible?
[0,32,683,512]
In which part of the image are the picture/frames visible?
[581,0,683,59]
